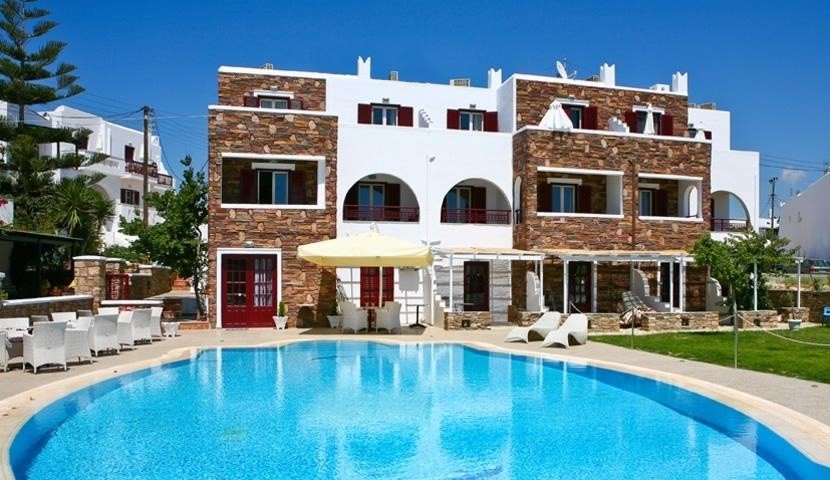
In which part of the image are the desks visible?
[406,304,427,329]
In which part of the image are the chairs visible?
[0,306,164,374]
[504,311,561,343]
[540,313,588,349]
[822,307,830,327]
[340,301,402,334]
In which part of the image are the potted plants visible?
[780,291,802,332]
[327,302,340,329]
[272,301,289,330]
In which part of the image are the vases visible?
[161,322,180,338]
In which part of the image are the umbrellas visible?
[291,226,434,308]
